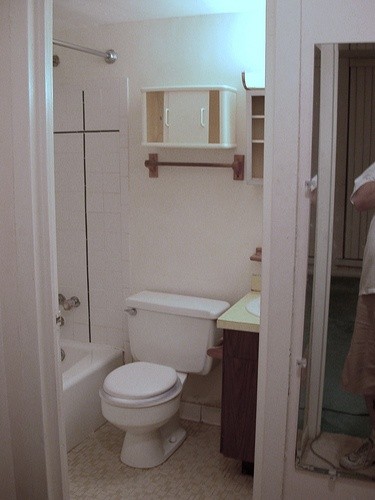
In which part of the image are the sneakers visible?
[340,437,375,470]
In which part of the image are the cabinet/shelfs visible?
[207,330,259,476]
[140,85,237,149]
[241,71,264,187]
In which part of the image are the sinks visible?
[245,294,260,317]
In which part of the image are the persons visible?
[340,161,375,473]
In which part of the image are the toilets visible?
[98,290,230,470]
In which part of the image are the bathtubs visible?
[60,338,124,452]
